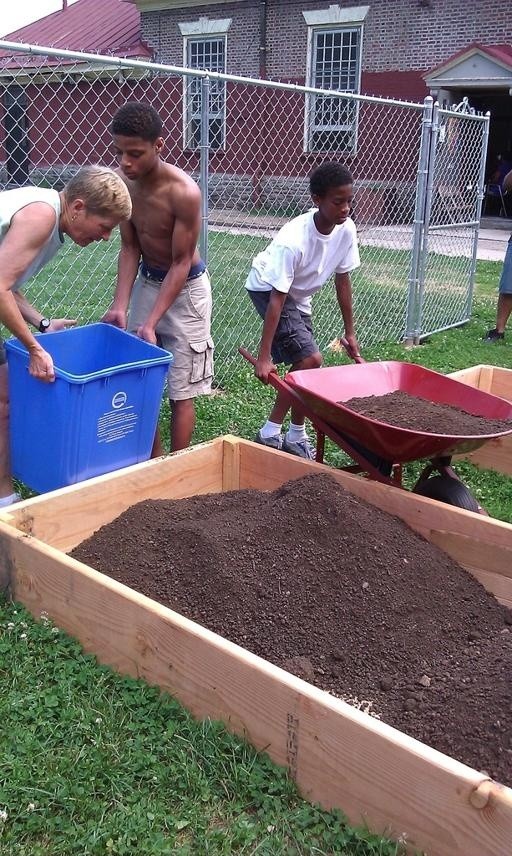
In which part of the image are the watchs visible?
[38,316,52,332]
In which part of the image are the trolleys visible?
[239,336,511,513]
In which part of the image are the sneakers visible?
[255,428,283,450]
[485,328,505,340]
[282,433,330,466]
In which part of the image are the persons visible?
[485,148,511,198]
[482,167,512,342]
[2,165,134,384]
[102,98,215,456]
[244,161,363,459]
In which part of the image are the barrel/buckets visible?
[2,320,175,496]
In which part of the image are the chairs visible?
[436,176,510,225]
